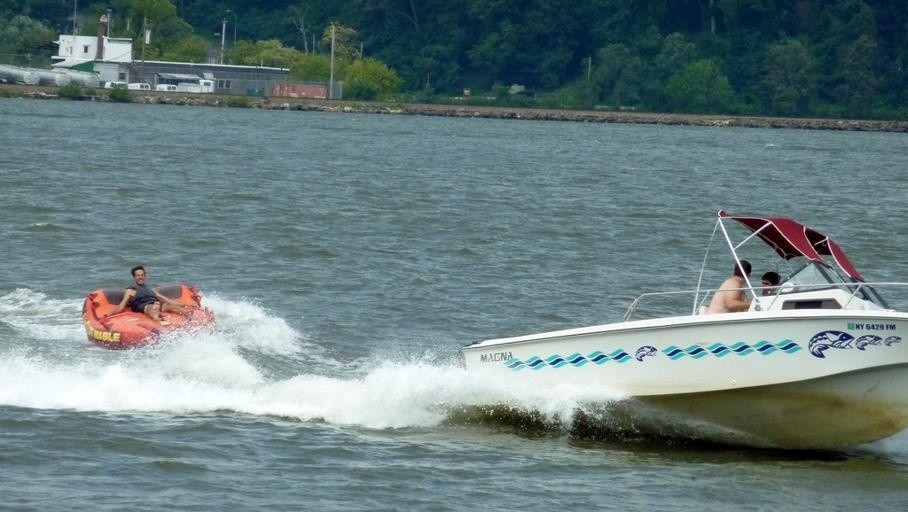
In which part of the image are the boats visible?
[461,210,908,451]
[82,284,217,350]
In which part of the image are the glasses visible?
[761,282,769,285]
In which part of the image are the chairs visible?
[697,305,709,315]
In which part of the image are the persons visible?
[701,257,760,313]
[99,263,197,327]
[756,272,781,293]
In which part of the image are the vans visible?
[110,82,177,92]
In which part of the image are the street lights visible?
[220,20,228,64]
